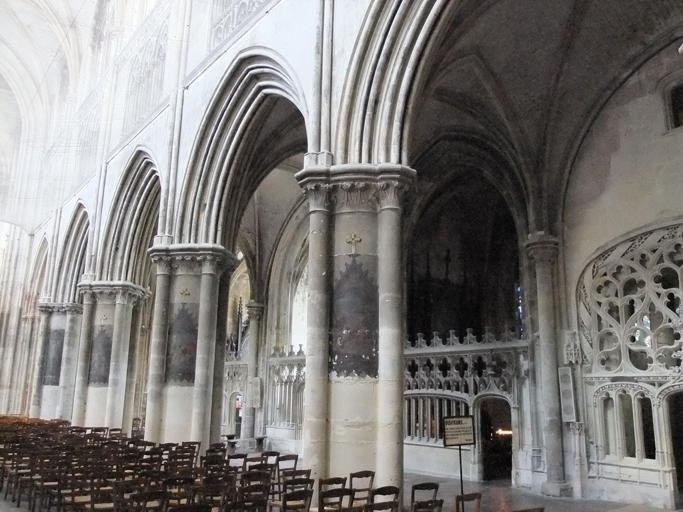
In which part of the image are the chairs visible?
[0,413,483,510]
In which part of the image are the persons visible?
[235,397,242,417]
